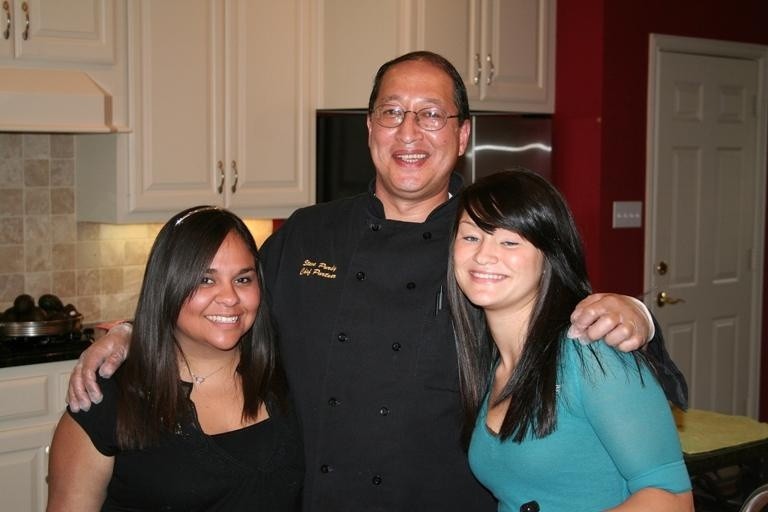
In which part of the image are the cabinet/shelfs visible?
[73,0,317,226]
[1,360,84,512]
[317,0,557,117]
[0,0,129,134]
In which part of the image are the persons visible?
[445,167,696,512]
[66,51,660,512]
[45,205,306,512]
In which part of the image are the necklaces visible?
[175,349,231,384]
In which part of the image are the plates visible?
[4,315,83,336]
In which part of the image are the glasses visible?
[370,104,459,131]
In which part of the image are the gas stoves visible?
[0,332,92,371]
